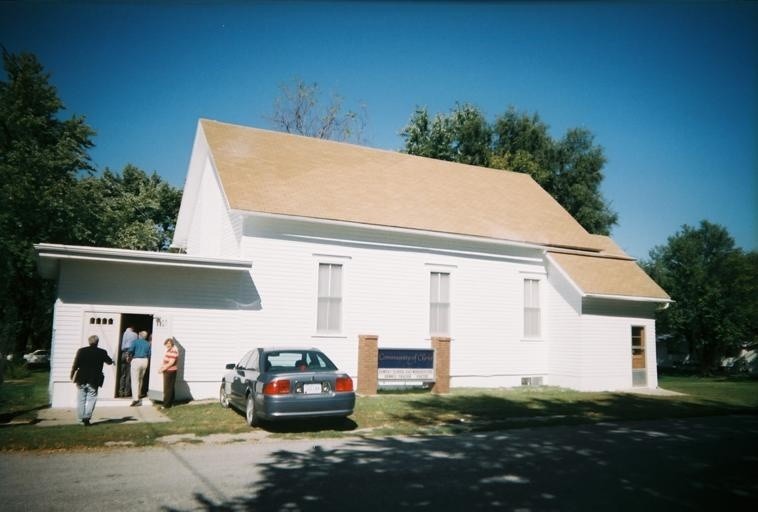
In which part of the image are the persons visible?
[124,330,150,408]
[158,338,179,410]
[69,335,114,425]
[117,323,139,397]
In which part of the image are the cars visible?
[28,350,51,364]
[220,348,354,426]
[7,352,29,361]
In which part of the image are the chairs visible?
[295,359,308,368]
[268,359,271,368]
